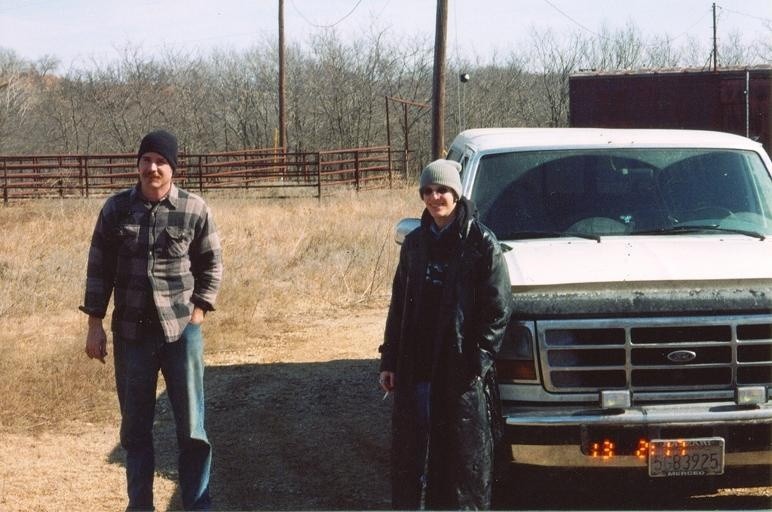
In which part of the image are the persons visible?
[378,159,514,512]
[79,130,223,512]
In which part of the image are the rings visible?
[379,378,386,384]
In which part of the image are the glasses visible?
[422,187,450,195]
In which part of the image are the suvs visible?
[394,127,772,478]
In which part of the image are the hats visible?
[137,131,177,170]
[420,158,463,201]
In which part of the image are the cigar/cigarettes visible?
[382,388,390,401]
